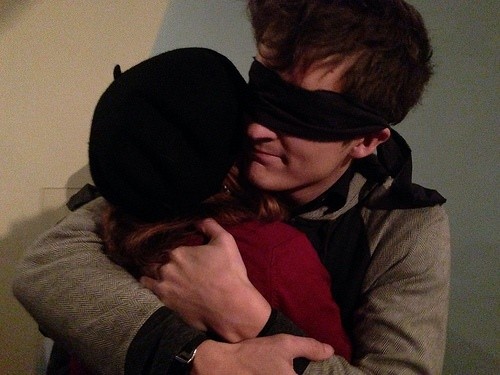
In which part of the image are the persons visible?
[10,0,450,375]
[46,46,356,374]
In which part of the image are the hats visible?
[88,47,249,222]
